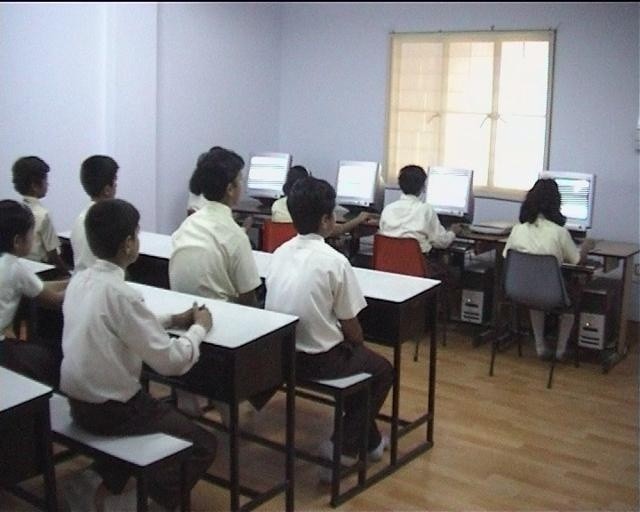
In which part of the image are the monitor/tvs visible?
[335,161,386,221]
[538,170,596,246]
[424,166,474,226]
[246,152,293,211]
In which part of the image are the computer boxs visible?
[577,276,624,351]
[461,259,494,325]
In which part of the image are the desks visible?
[0,365,65,512]
[0,248,306,508]
[51,224,442,469]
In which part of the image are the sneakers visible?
[536,343,553,361]
[369,435,390,462]
[63,494,99,512]
[175,387,200,419]
[102,494,141,512]
[329,441,359,467]
[211,398,248,431]
[556,343,576,363]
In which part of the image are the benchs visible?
[40,380,197,508]
[296,366,378,506]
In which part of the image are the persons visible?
[12,156,69,272]
[186,146,254,233]
[378,165,463,278]
[169,150,287,433]
[265,178,395,466]
[68,155,119,273]
[270,166,373,237]
[502,177,594,363]
[0,199,64,395]
[58,198,219,511]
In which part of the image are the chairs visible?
[489,244,582,388]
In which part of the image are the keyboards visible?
[449,241,472,250]
[561,259,600,271]
